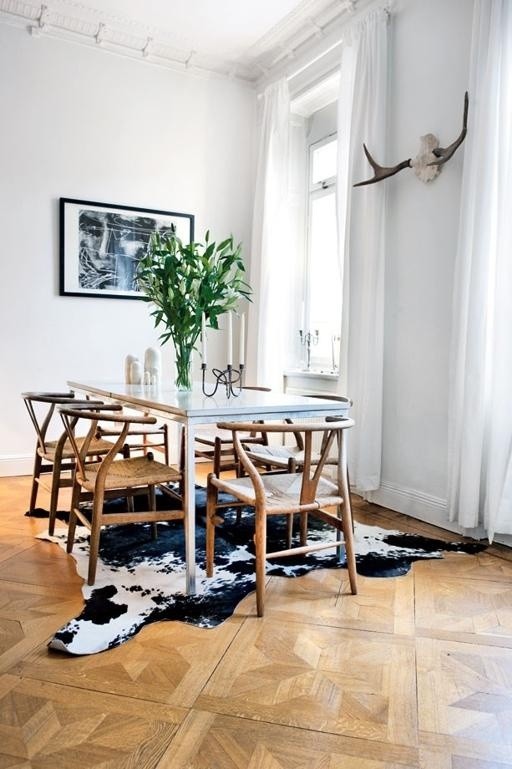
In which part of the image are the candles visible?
[224,308,233,368]
[238,311,246,368]
[301,302,305,332]
[201,308,208,369]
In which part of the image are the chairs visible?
[20,389,138,537]
[205,414,356,619]
[193,382,272,529]
[233,387,357,562]
[52,403,185,587]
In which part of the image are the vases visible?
[174,359,193,390]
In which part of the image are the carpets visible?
[24,480,488,658]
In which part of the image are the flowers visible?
[133,219,254,381]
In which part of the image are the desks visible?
[63,372,353,596]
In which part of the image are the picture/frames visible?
[57,195,196,303]
